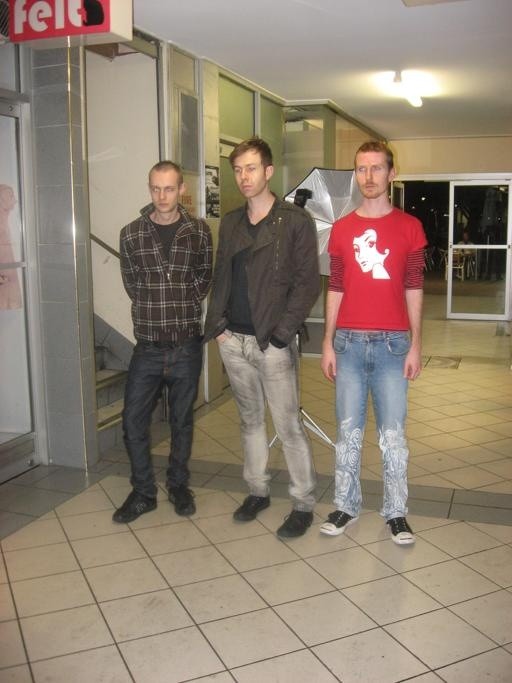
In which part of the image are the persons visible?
[319,140,428,547]
[352,227,391,281]
[110,158,214,525]
[458,232,477,252]
[198,134,320,542]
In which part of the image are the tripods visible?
[268,331,336,448]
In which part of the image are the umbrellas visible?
[283,165,364,278]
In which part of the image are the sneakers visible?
[110,487,159,525]
[274,507,315,539]
[386,515,416,545]
[166,483,198,516]
[318,508,360,536]
[232,493,272,522]
